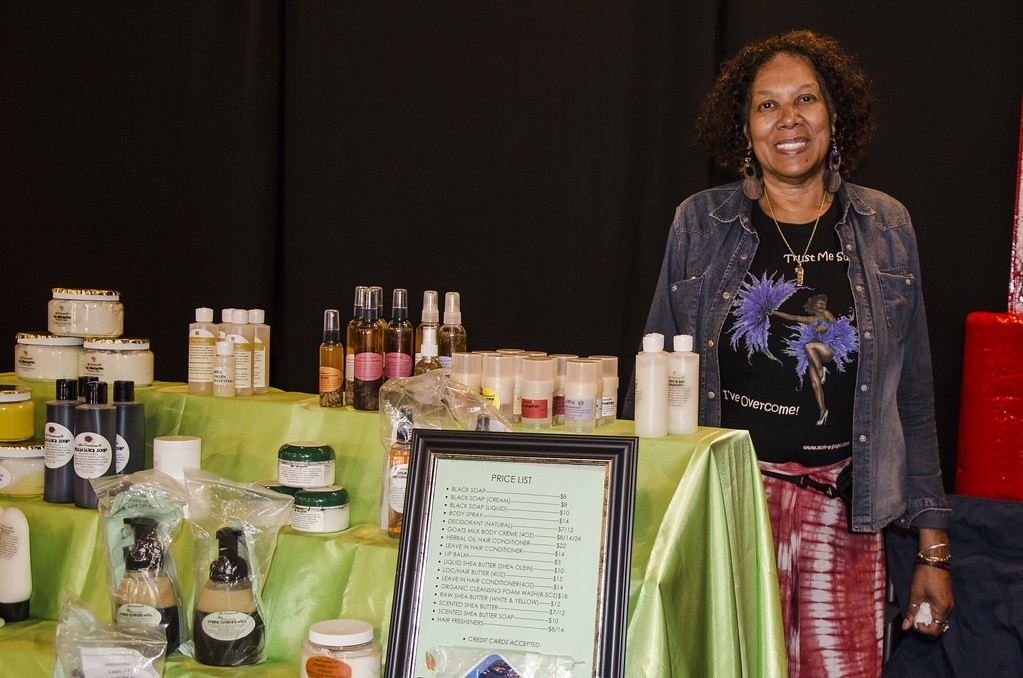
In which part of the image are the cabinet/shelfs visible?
[0,372,786,678]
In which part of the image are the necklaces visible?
[763,186,828,286]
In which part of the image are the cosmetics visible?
[112,514,181,656]
[187,307,270,400]
[299,619,384,677]
[152,433,202,520]
[318,279,618,436]
[41,373,147,510]
[15,288,155,390]
[382,406,416,540]
[243,440,353,535]
[0,506,33,623]
[634,331,699,434]
[192,528,269,669]
[0,388,47,499]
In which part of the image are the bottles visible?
[44,376,145,509]
[0,439,44,498]
[387,408,414,538]
[301,620,381,678]
[188,307,270,396]
[251,442,348,533]
[15,333,154,387]
[452,349,619,432]
[0,384,36,442]
[666,335,699,434]
[320,309,344,407]
[48,288,124,337]
[346,286,467,411]
[636,333,669,438]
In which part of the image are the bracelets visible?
[918,543,952,571]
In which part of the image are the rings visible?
[941,619,950,633]
[934,619,941,624]
[909,602,920,608]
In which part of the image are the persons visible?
[619,29,958,678]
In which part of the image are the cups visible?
[153,436,201,519]
